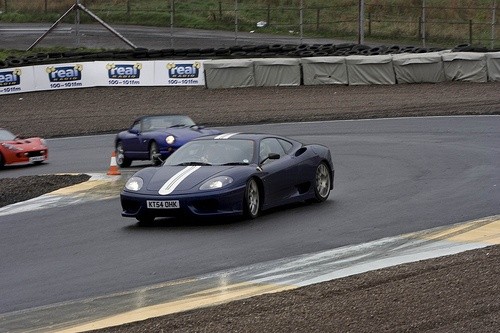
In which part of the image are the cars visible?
[120,131,335,224]
[0,126,49,168]
[114,113,222,165]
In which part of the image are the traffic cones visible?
[106,150,124,177]
